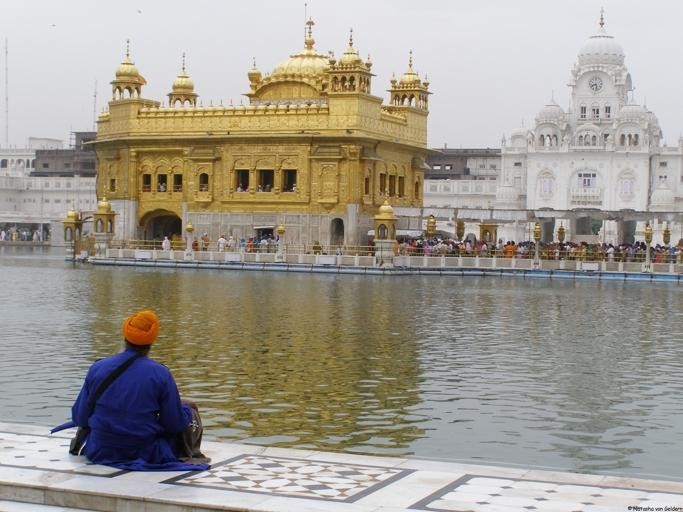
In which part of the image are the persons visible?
[192,233,279,253]
[162,236,170,251]
[314,240,321,254]
[237,183,249,192]
[284,183,295,192]
[396,234,683,264]
[72,312,211,471]
[322,244,327,254]
[258,183,274,192]
[158,182,166,192]
[336,246,343,256]
[0,223,51,242]
[368,236,376,256]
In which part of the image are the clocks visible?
[588,77,603,92]
